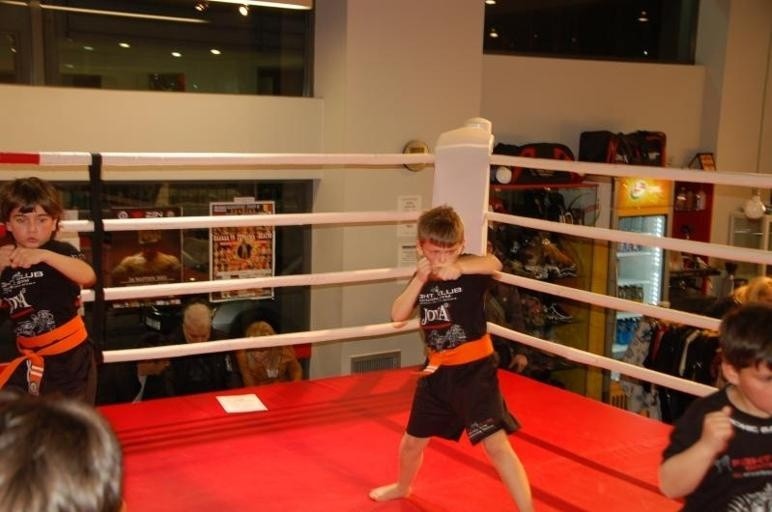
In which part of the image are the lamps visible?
[238,4,257,17]
[744,18,772,219]
[192,0,211,16]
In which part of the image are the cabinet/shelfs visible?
[485,182,598,396]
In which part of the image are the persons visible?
[656,300,772,512]
[0,176,97,411]
[74,302,305,403]
[109,230,182,285]
[0,388,124,512]
[729,274,772,309]
[369,203,533,512]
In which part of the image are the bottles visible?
[617,225,650,348]
[717,261,737,298]
[676,184,708,210]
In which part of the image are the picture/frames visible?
[350,349,401,373]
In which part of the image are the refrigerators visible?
[583,170,673,411]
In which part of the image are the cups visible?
[732,278,747,291]
